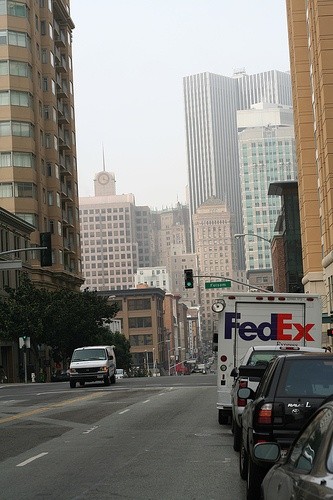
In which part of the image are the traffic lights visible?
[326,328,333,337]
[183,269,194,289]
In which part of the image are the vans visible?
[67,345,116,388]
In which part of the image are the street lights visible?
[153,347,156,368]
[145,350,150,378]
[168,346,182,376]
[156,339,171,365]
[234,233,275,293]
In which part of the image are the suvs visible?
[229,346,333,452]
[236,351,333,500]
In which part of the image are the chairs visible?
[255,360,269,366]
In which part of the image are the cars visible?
[183,357,217,375]
[253,393,333,500]
[114,369,126,380]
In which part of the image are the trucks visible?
[212,291,323,426]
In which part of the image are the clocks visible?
[98,174,110,185]
